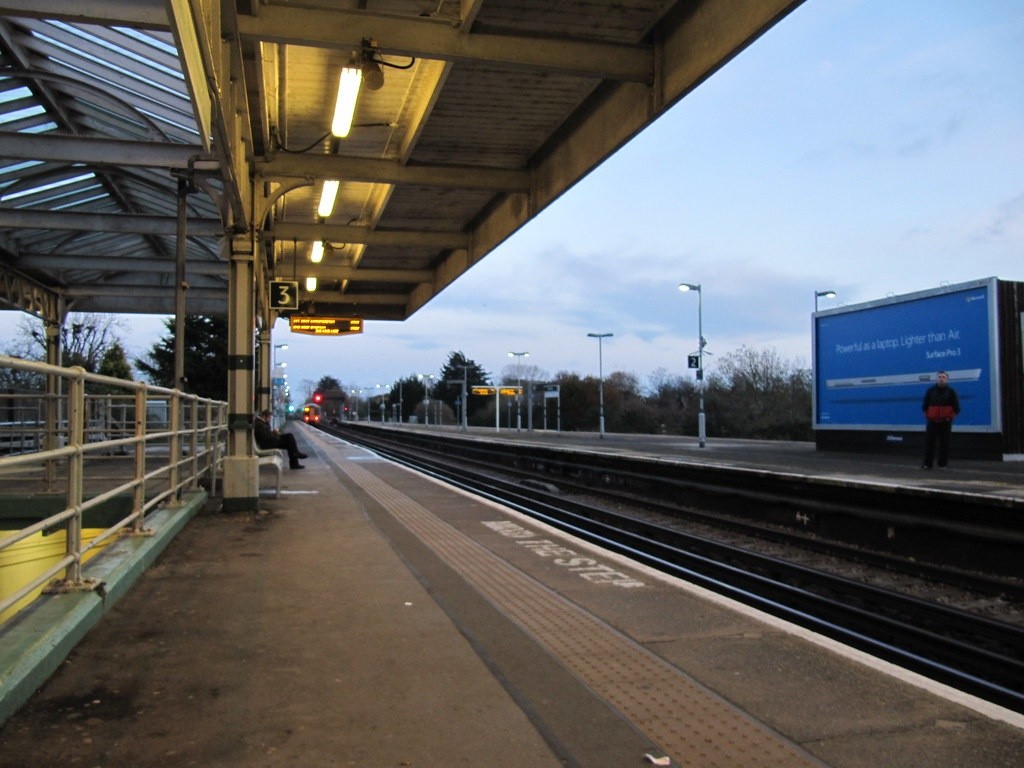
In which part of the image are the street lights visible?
[376,384,390,425]
[507,351,529,432]
[458,365,479,430]
[351,389,363,422]
[678,283,705,448]
[418,373,434,426]
[587,333,614,440]
[274,343,288,370]
[815,290,836,312]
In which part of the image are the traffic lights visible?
[289,405,294,411]
[344,405,348,411]
[314,394,321,402]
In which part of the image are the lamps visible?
[331,60,363,140]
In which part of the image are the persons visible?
[254,408,308,469]
[918,371,960,470]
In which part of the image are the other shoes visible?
[293,451,307,458]
[924,463,933,468]
[938,464,946,471]
[290,463,305,469]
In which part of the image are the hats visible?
[262,409,274,416]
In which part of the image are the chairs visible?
[258,455,284,499]
[254,438,283,458]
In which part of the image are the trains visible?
[296,403,321,423]
